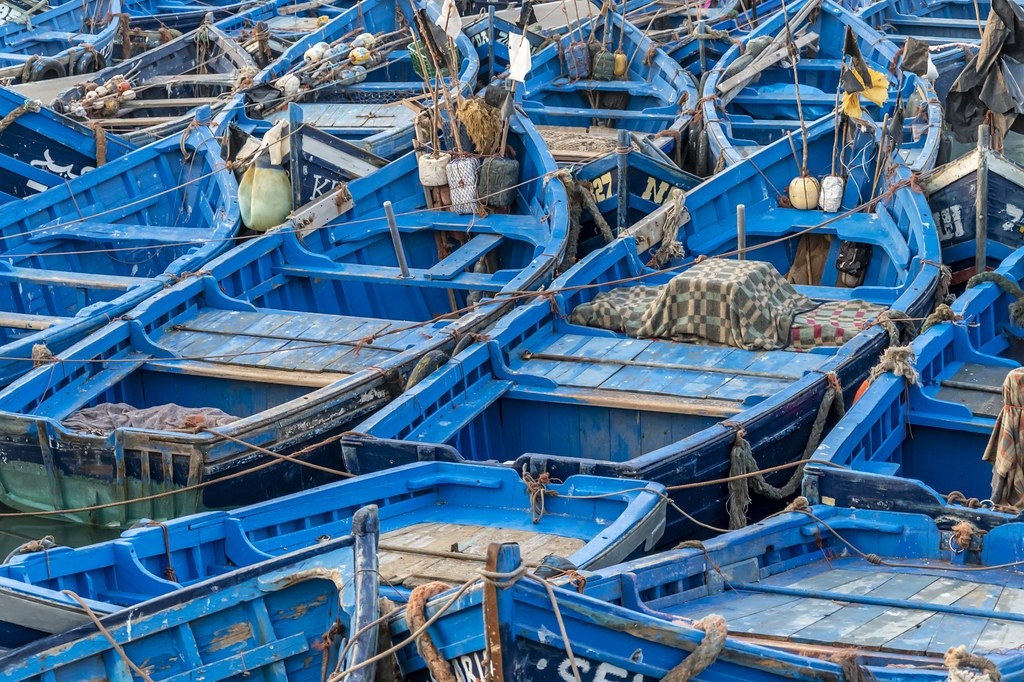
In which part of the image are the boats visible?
[0,461,667,679]
[476,499,1023,682]
[0,0,1024,548]
